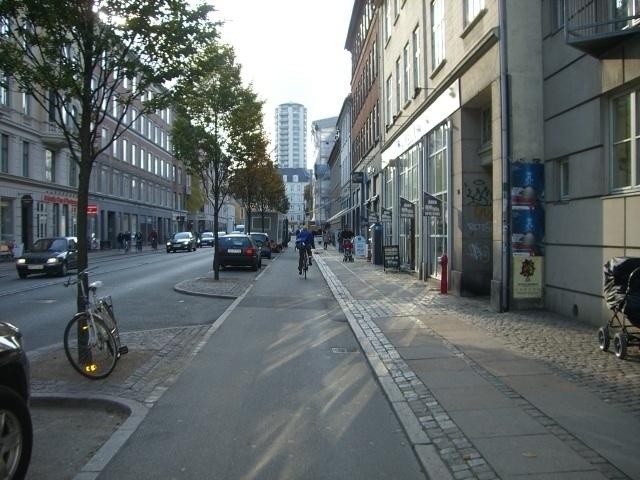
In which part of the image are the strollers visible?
[342,238,354,262]
[598,256,640,359]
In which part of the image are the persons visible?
[295,226,315,275]
[295,228,301,236]
[322,229,329,249]
[118,230,158,252]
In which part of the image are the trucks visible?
[245,210,288,253]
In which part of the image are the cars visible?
[166,225,271,271]
[0,321,34,480]
[16,236,77,278]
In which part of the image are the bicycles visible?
[299,248,312,279]
[124,238,158,255]
[63,265,128,379]
